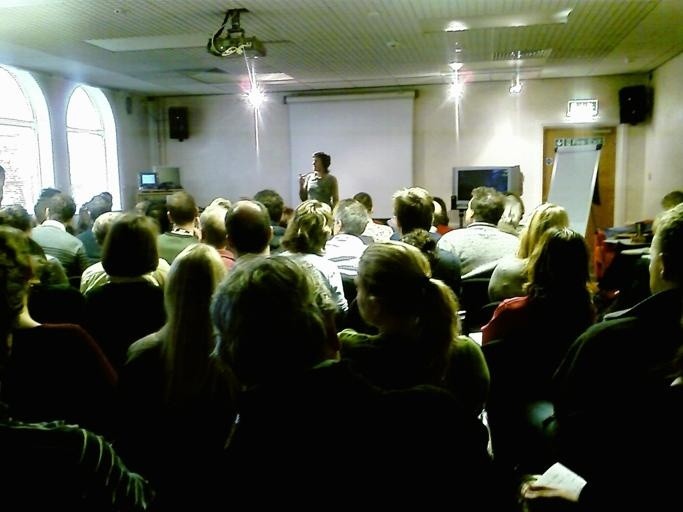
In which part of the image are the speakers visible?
[168,106,188,139]
[618,85,646,124]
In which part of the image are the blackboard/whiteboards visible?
[546,143,603,239]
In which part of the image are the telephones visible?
[158,181,182,189]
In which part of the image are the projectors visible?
[206,37,266,59]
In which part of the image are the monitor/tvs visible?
[139,173,156,187]
[451,166,523,209]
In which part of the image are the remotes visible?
[451,196,456,210]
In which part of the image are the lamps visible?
[508,71,525,98]
[446,60,467,106]
[244,57,267,112]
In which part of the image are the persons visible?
[297,152,339,210]
[1,187,683,511]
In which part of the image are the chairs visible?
[343,277,509,335]
